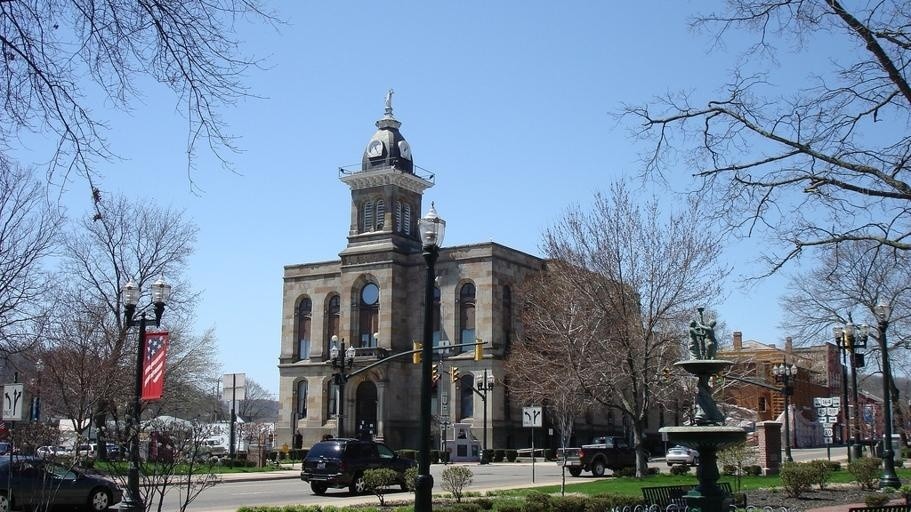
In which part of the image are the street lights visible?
[770,362,800,462]
[35,357,45,424]
[872,295,905,493]
[830,319,872,460]
[114,272,174,512]
[29,377,36,422]
[409,194,449,512]
[476,369,495,462]
[330,333,357,438]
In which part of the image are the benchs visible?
[849,505,911,512]
[641,482,747,512]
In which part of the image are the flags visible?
[141,331,168,399]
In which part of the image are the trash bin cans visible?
[883,433,902,460]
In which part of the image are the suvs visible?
[299,433,418,497]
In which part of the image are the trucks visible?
[189,437,228,462]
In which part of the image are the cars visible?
[850,433,911,446]
[663,443,701,467]
[0,441,125,511]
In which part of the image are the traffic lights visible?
[413,341,424,366]
[450,366,460,384]
[432,363,439,383]
[661,368,670,383]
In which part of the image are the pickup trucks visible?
[554,434,653,478]
